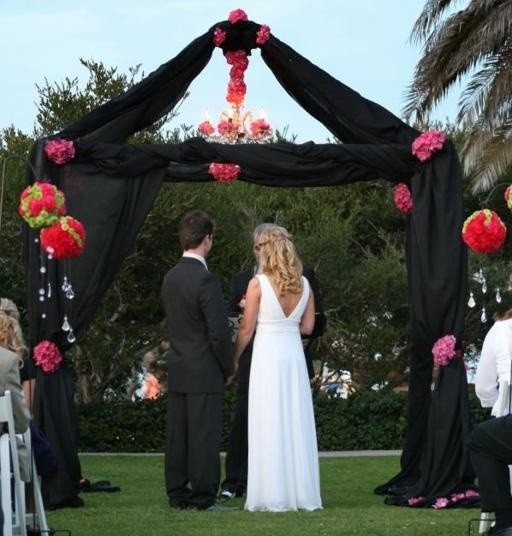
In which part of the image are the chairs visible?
[0,390,50,536]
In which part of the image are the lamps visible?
[199,5,277,147]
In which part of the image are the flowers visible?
[19,137,88,374]
[429,333,458,368]
[206,160,241,184]
[393,127,512,254]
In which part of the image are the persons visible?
[161,211,241,512]
[469,276,512,536]
[0,298,36,536]
[219,266,326,501]
[228,223,324,512]
